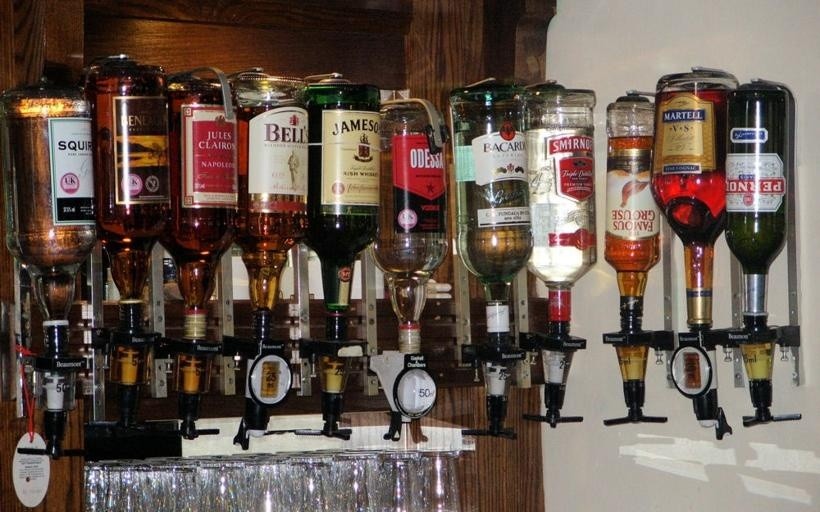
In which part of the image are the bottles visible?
[603,97,663,333]
[300,84,381,335]
[224,76,311,341]
[449,88,536,348]
[83,66,180,332]
[76,459,458,512]
[158,80,242,343]
[648,68,737,324]
[3,86,101,352]
[368,102,451,353]
[525,89,597,335]
[722,82,791,317]
